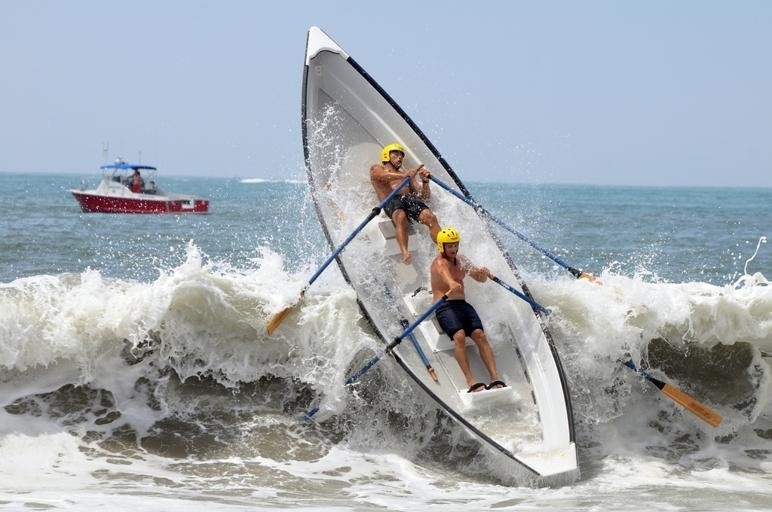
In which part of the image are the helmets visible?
[380,143,406,165]
[436,227,461,253]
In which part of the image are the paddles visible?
[488,274,722,427]
[424,172,594,282]
[303,289,452,420]
[267,164,424,336]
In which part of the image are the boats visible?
[71,170,210,217]
[302,22,584,492]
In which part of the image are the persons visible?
[430,228,503,392]
[369,143,442,265]
[129,168,155,194]
